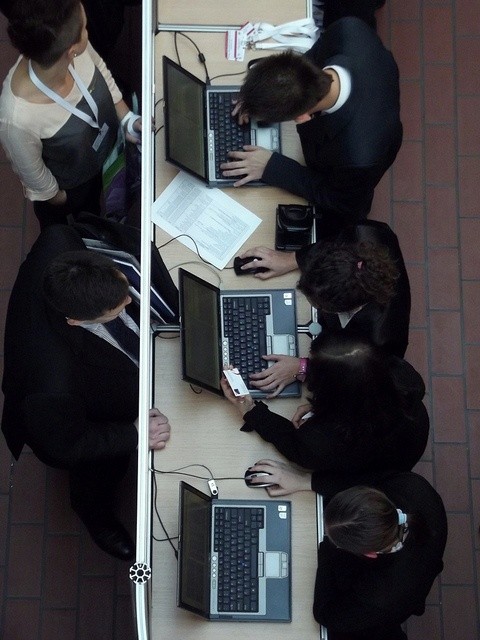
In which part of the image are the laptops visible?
[167,263,313,407]
[159,56,286,190]
[158,479,298,627]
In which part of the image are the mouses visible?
[233,450,289,501]
[228,244,279,283]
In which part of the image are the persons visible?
[219,326,431,473]
[240,217,413,400]
[0,0,159,234]
[0,223,171,560]
[219,12,404,223]
[248,459,449,639]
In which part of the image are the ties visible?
[103,316,140,361]
